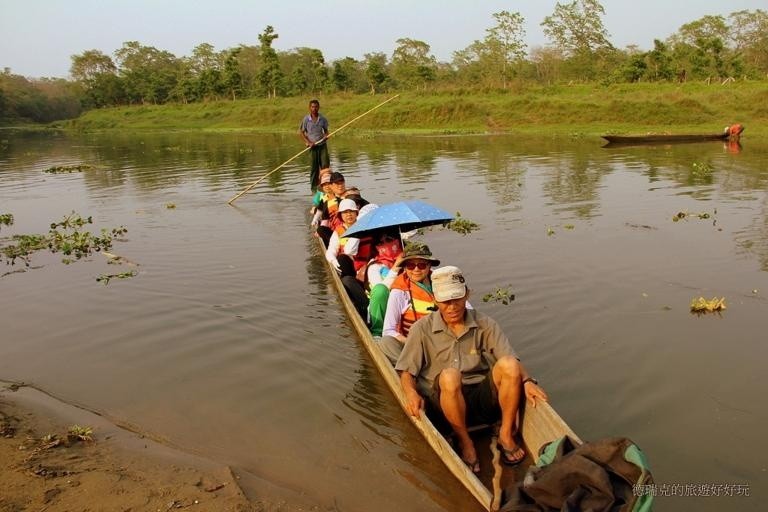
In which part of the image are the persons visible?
[395,265,549,476]
[365,228,406,343]
[309,167,378,322]
[299,99,331,197]
[377,240,478,368]
[724,124,744,136]
[723,136,742,154]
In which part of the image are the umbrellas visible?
[339,198,456,249]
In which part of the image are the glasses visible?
[405,262,429,270]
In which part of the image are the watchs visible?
[524,377,538,386]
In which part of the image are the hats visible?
[395,241,441,266]
[724,126,730,134]
[339,187,370,204]
[330,171,345,183]
[431,265,468,303]
[336,199,360,213]
[357,204,381,221]
[316,173,332,193]
[318,168,332,179]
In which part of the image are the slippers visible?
[459,443,482,476]
[496,438,525,465]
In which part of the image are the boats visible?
[601,132,731,146]
[310,191,583,510]
